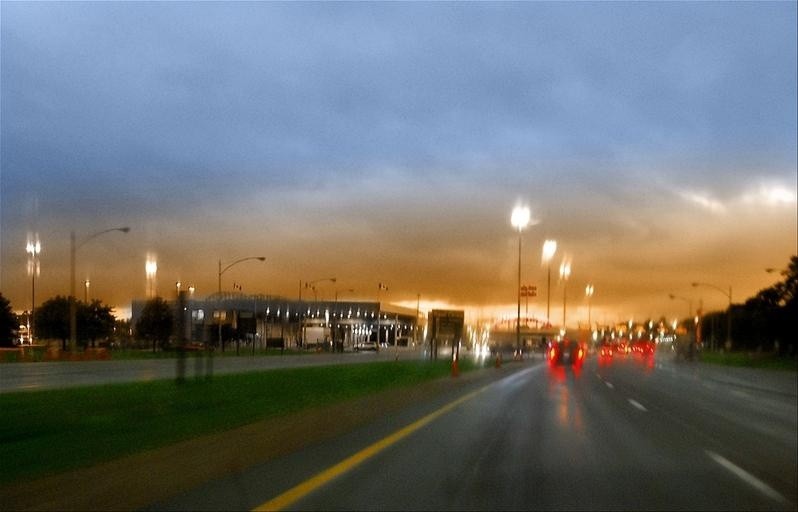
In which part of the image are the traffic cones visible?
[495,357,501,369]
[451,360,458,377]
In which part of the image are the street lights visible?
[585,283,594,334]
[511,204,531,363]
[70,226,131,358]
[218,255,267,355]
[557,259,571,330]
[541,237,556,330]
[298,276,337,354]
[333,287,355,355]
[518,282,536,328]
[25,240,41,345]
[670,280,733,355]
